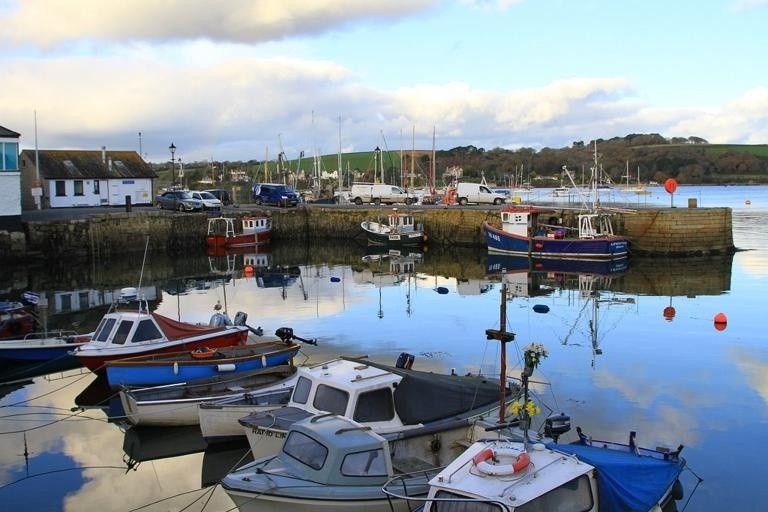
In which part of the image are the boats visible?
[485,257,637,372]
[356,248,429,275]
[357,208,429,247]
[204,248,273,273]
[207,216,274,248]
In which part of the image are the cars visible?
[154,189,231,211]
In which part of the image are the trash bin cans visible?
[126,195,131,212]
[688,198,697,209]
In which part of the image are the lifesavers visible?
[475,445,530,477]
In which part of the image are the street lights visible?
[167,141,180,215]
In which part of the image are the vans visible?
[350,185,418,204]
[258,267,301,288]
[254,183,302,208]
[456,183,508,206]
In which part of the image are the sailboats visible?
[483,134,652,256]
[304,110,340,205]
